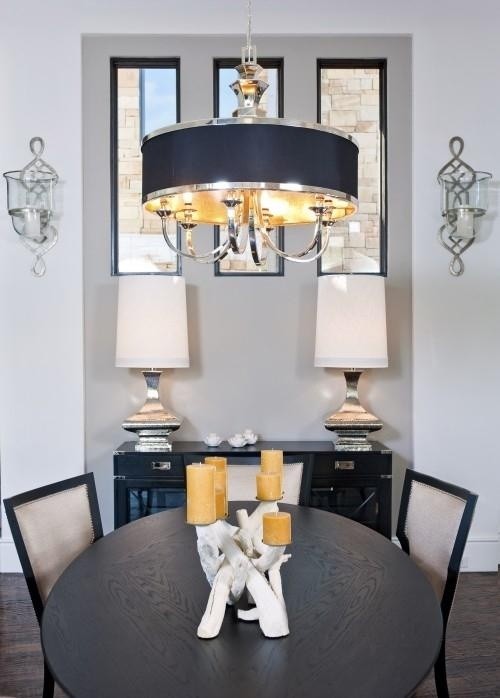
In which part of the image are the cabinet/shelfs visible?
[113,442,391,544]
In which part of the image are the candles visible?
[186,447,295,547]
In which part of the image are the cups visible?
[203,433,223,447]
[240,430,258,445]
[228,434,247,448]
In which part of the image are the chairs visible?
[395,468,477,695]
[1,471,106,696]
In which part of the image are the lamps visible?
[437,136,491,277]
[2,136,60,277]
[117,276,188,451]
[316,274,387,452]
[140,3,355,273]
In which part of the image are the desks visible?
[42,501,445,698]
